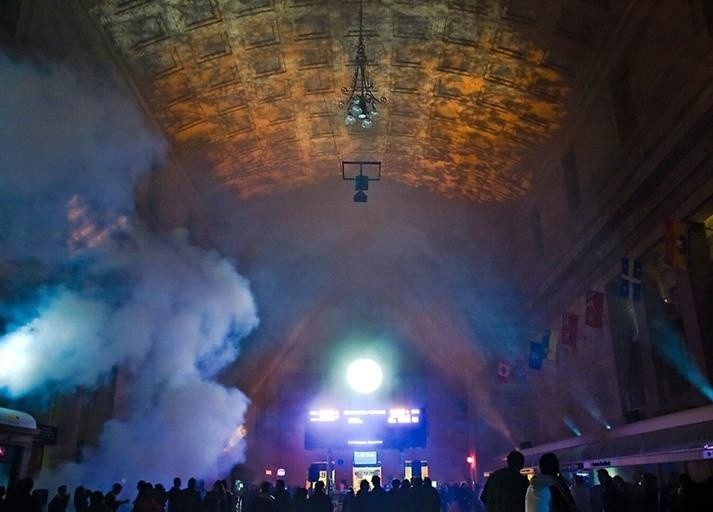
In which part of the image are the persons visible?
[1,450,712,511]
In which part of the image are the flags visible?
[656,218,691,274]
[615,254,643,303]
[582,289,605,329]
[493,311,579,384]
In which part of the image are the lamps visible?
[336,1,386,130]
[341,160,381,203]
